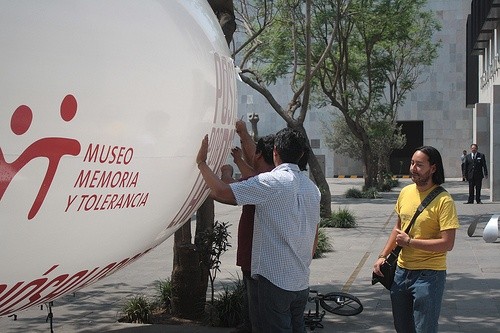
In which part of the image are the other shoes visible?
[477,202,483,204]
[463,202,474,204]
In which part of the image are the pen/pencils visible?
[236,115,244,130]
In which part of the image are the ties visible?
[473,153,475,159]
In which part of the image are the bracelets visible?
[198,160,207,169]
[407,237,412,248]
[377,254,387,259]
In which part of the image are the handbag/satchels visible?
[372,259,397,290]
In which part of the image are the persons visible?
[463,144,488,204]
[459,150,469,182]
[197,112,321,333]
[371,146,460,333]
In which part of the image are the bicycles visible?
[302,289,364,330]
